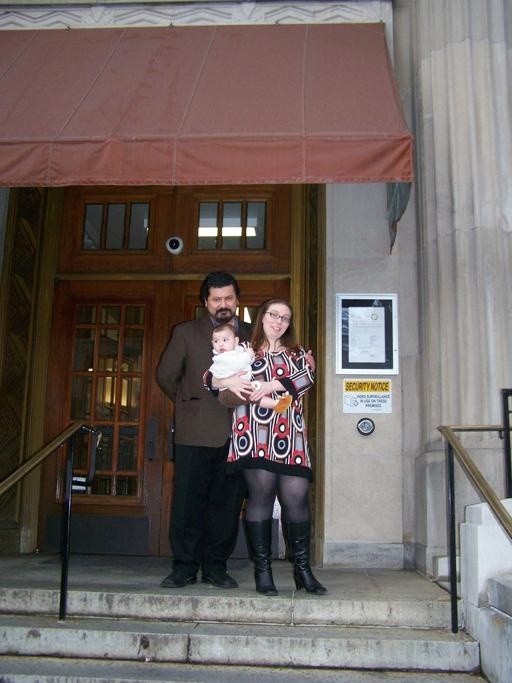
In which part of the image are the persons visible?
[153,270,316,590]
[208,323,295,416]
[201,296,329,597]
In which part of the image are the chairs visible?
[272,395,294,415]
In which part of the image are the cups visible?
[264,309,291,323]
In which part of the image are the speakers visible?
[242,516,279,595]
[281,517,329,595]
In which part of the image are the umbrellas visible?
[158,568,198,588]
[200,568,240,589]
